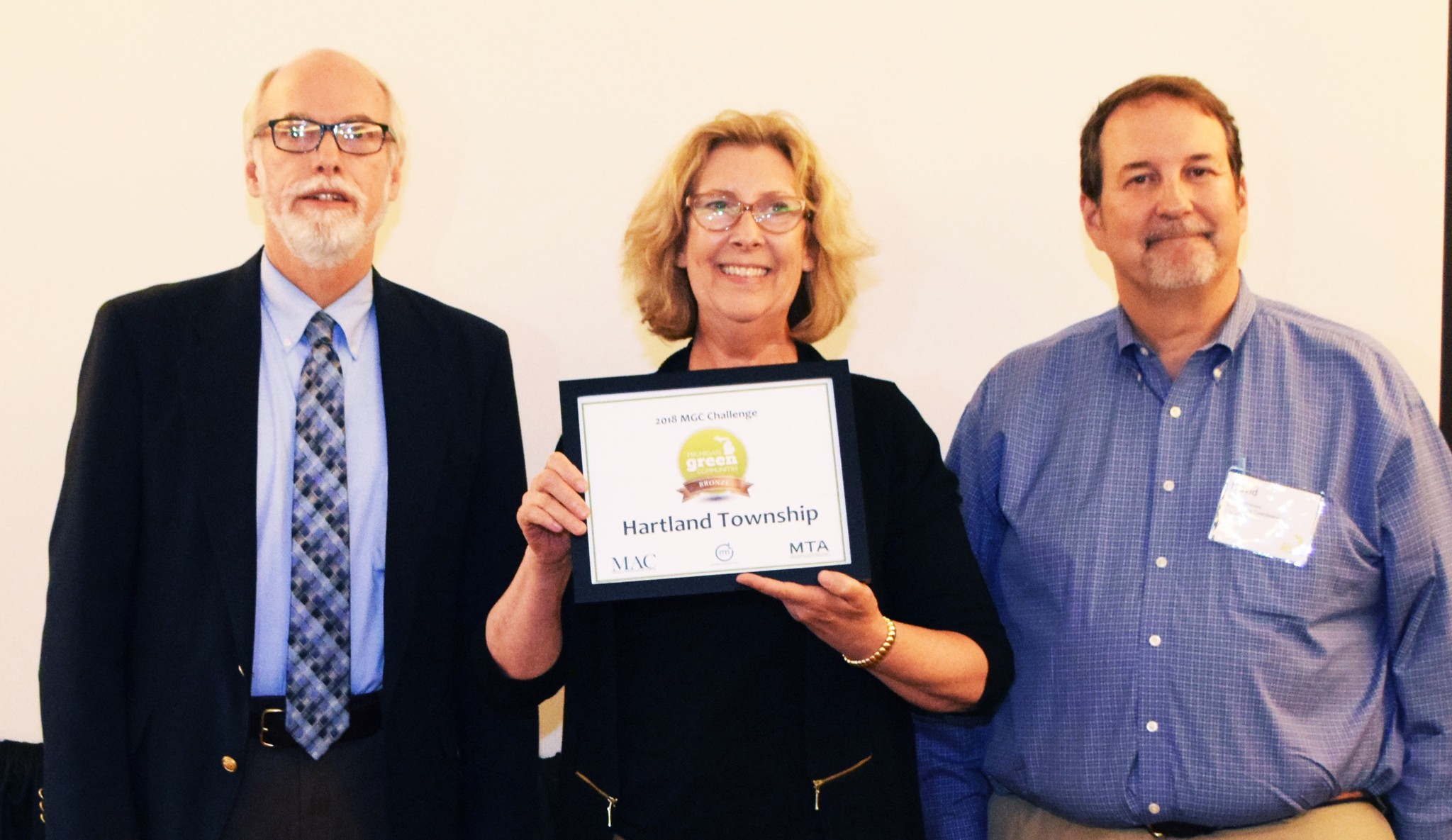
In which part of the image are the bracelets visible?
[841,616,897,668]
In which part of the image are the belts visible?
[252,700,286,751]
[1147,788,1371,840]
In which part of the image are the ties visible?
[286,315,351,759]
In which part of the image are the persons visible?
[915,76,1452,840]
[486,111,1015,840]
[37,49,540,840]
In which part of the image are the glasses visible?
[684,190,817,234]
[255,116,396,155]
